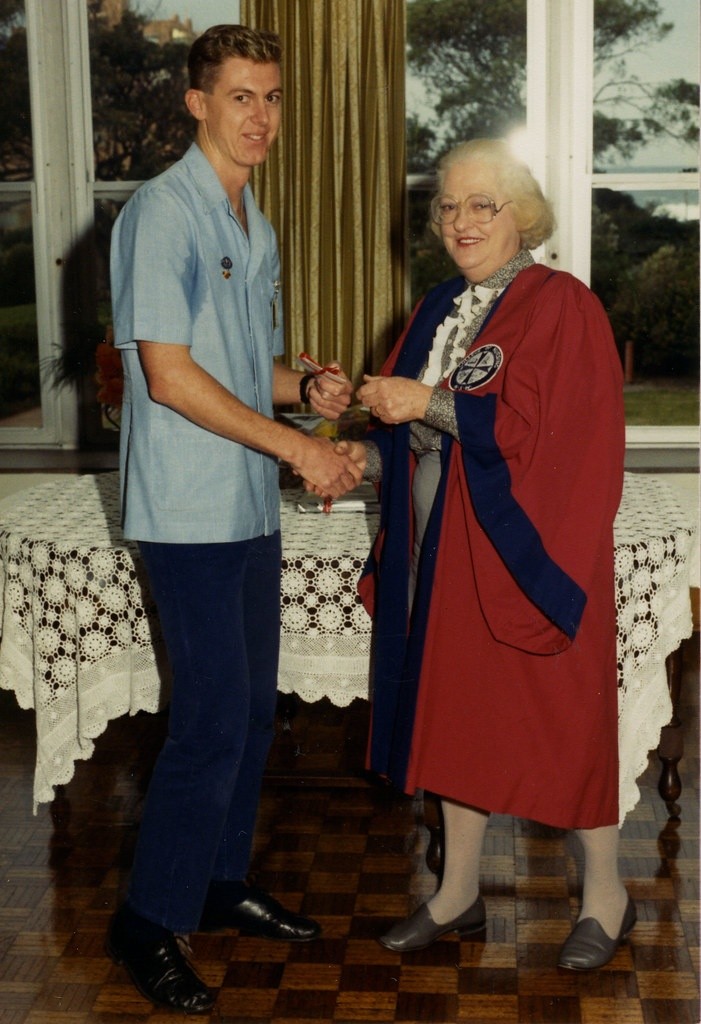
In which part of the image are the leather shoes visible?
[556,884,638,972]
[100,905,217,1016]
[196,879,324,947]
[376,893,489,955]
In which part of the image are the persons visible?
[294,140,639,971]
[106,24,363,1015]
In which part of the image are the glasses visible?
[431,193,514,226]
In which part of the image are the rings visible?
[375,405,386,416]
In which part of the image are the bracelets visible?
[300,374,315,404]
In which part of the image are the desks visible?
[0,468,701,876]
